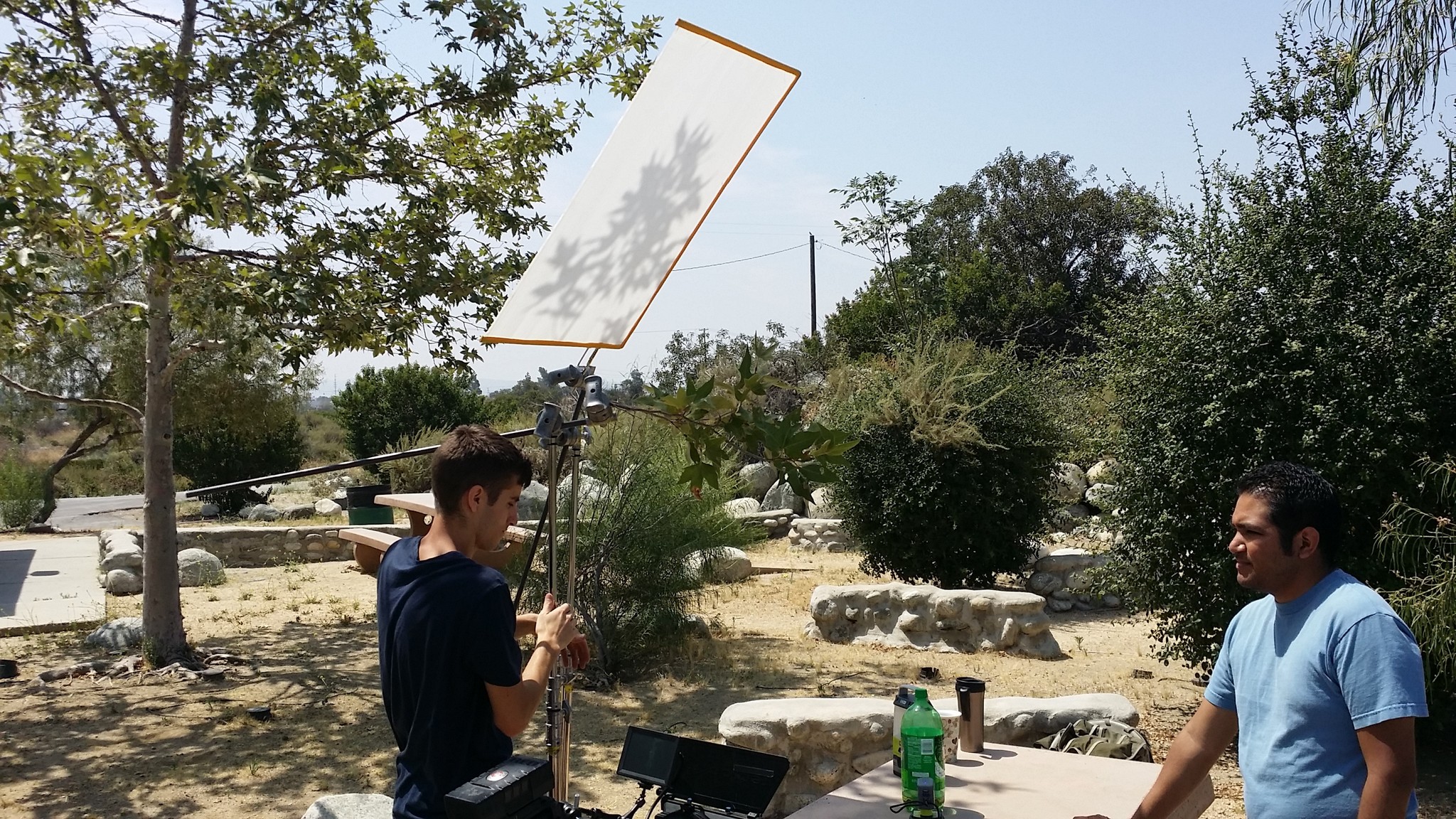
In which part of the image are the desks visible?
[785,739,1215,819]
[374,493,435,537]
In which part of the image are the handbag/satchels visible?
[1033,714,1154,764]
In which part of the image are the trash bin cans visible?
[347,483,393,525]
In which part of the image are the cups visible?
[937,710,963,765]
[955,677,987,753]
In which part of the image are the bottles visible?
[900,688,946,812]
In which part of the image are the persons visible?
[1073,462,1428,819]
[377,424,590,819]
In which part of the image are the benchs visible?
[338,526,547,574]
